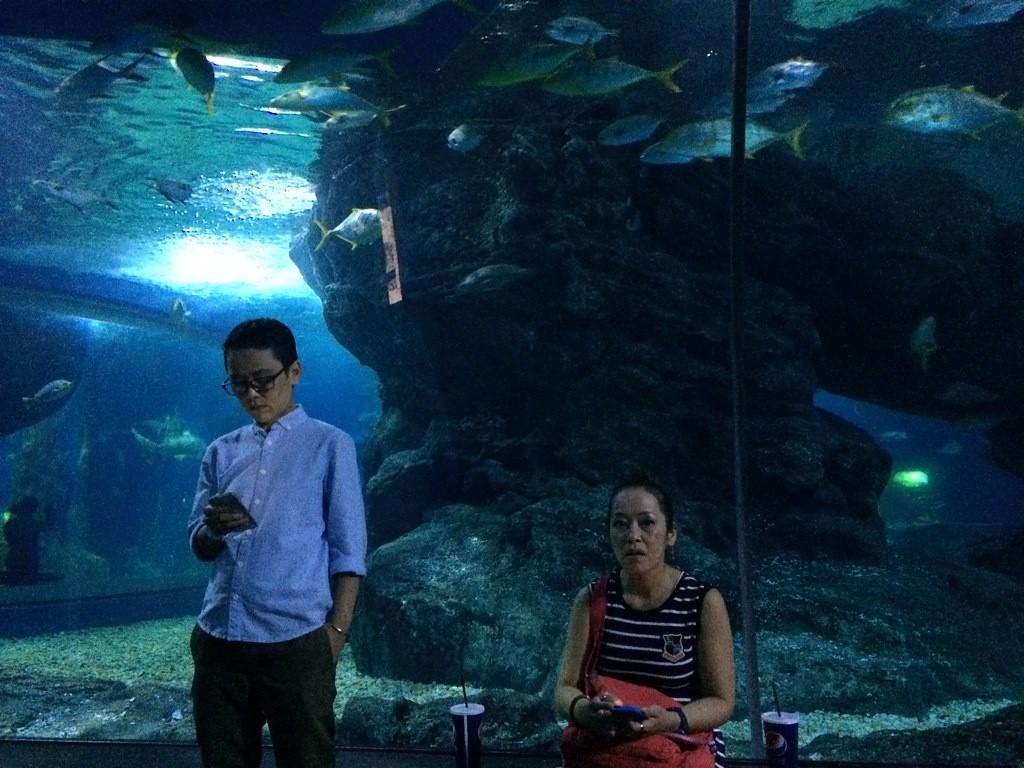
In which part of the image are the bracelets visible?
[329,618,350,638]
[569,691,588,727]
[202,524,231,543]
[666,703,691,735]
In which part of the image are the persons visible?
[180,316,370,768]
[549,474,739,768]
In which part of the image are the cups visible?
[762,711,799,768]
[450,703,485,768]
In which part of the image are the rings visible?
[637,721,646,732]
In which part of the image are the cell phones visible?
[209,492,256,533]
[610,705,645,723]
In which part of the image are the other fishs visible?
[456,264,537,293]
[54,53,151,100]
[22,380,75,410]
[30,179,122,217]
[257,0,1024,165]
[130,424,208,461]
[141,176,193,206]
[169,47,216,117]
[312,208,391,254]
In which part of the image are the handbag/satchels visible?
[562,676,717,768]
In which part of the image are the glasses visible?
[221,359,296,396]
